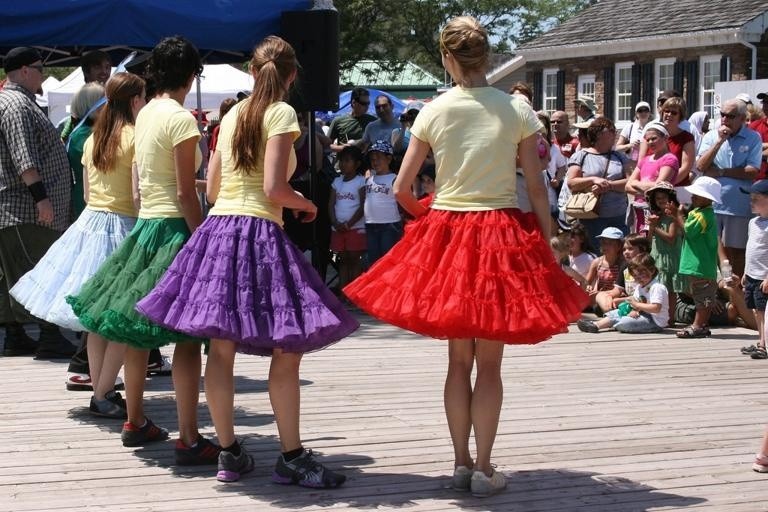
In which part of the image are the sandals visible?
[742,343,759,354]
[678,326,710,337]
[752,345,768,359]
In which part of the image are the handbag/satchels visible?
[566,191,602,219]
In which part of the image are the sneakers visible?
[577,319,598,333]
[453,465,470,492]
[470,463,508,498]
[753,450,767,474]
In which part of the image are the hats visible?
[684,177,723,205]
[596,227,623,240]
[549,205,573,232]
[644,182,680,209]
[574,91,768,137]
[368,140,392,154]
[4,47,42,71]
[739,180,768,194]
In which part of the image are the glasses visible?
[31,64,43,72]
[357,99,370,105]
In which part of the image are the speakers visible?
[281,9,340,113]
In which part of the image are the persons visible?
[190,88,253,221]
[509,81,768,361]
[343,15,592,497]
[135,36,360,490]
[752,424,768,473]
[1,39,222,468]
[283,88,436,304]
